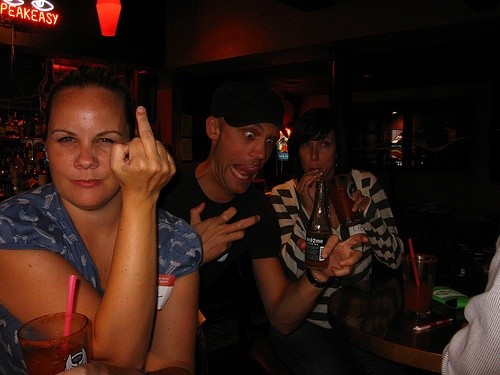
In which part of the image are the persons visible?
[0,64,202,375]
[158,82,500,375]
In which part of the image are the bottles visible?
[303,177,329,270]
[1,112,49,192]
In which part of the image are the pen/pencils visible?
[413,318,454,331]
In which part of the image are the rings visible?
[364,196,369,200]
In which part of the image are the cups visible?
[326,173,363,228]
[18,312,90,375]
[402,254,432,315]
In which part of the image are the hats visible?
[211,81,288,138]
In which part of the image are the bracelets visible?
[305,268,327,288]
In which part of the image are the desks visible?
[328,257,494,372]
